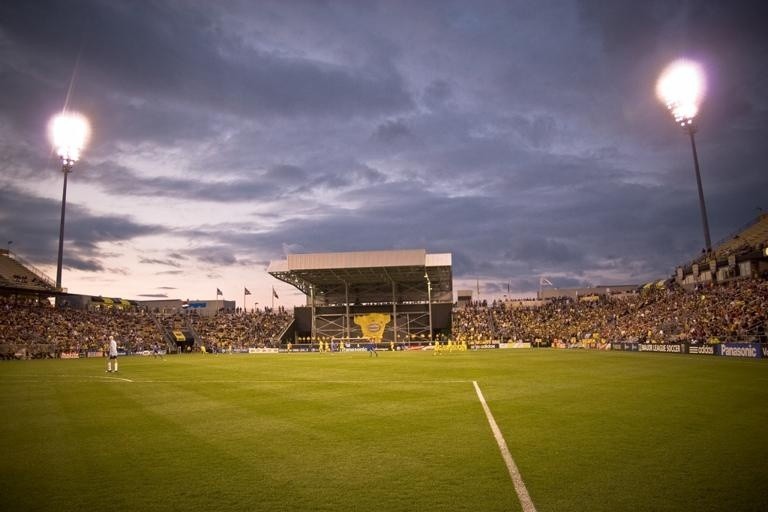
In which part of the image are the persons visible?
[105,335,119,372]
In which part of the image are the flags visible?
[8,241,12,244]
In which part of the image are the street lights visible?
[54,115,88,308]
[654,69,720,288]
